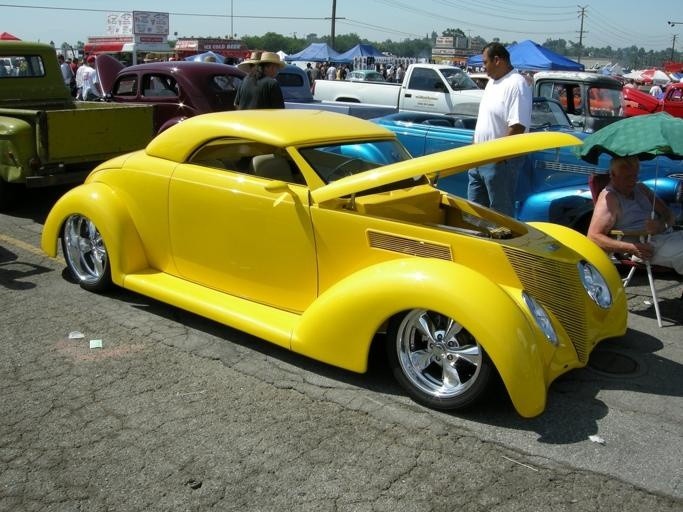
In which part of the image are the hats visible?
[254,51,286,71]
[237,51,262,74]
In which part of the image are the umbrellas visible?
[573,110,683,245]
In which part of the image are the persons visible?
[609,73,683,119]
[464,42,535,224]
[0,50,251,103]
[288,57,489,95]
[233,51,261,110]
[238,51,286,109]
[584,155,682,278]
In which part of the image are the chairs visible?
[158,89,177,96]
[587,174,682,328]
[251,153,297,184]
[423,120,452,127]
[453,117,477,130]
